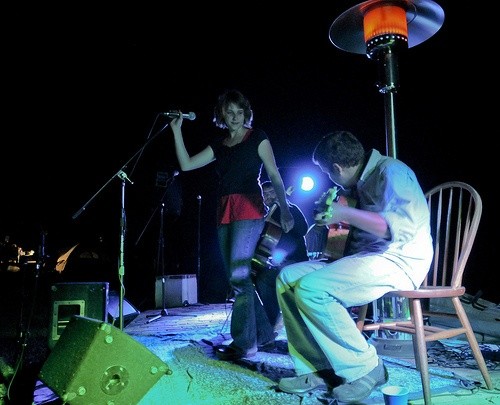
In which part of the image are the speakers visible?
[105,290,139,330]
[153,274,196,307]
[37,314,169,405]
[48,280,110,348]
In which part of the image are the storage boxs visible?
[155,274,198,309]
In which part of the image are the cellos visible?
[217,183,297,335]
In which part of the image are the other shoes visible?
[257,340,277,352]
[331,358,389,403]
[278,368,342,394]
[216,340,256,359]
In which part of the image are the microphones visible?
[161,111,196,121]
[196,194,202,199]
[165,171,179,184]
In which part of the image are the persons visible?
[260,180,308,329]
[171,91,293,358]
[276,131,434,402]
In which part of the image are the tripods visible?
[134,182,197,323]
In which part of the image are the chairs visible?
[356,182,493,405]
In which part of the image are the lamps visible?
[328,0,445,159]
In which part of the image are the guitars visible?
[319,191,358,258]
